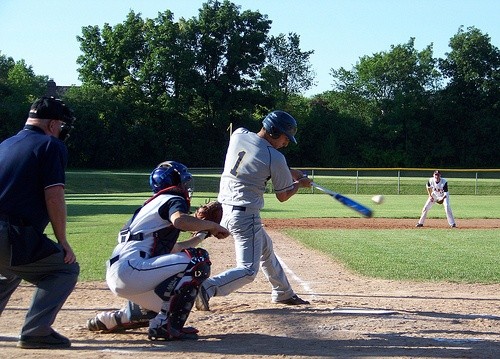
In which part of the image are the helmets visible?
[262,110,297,145]
[152,166,180,190]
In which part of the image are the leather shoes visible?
[17,332,71,348]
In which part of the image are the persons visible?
[416,170,456,227]
[86,160,230,340]
[194,109,313,311]
[0,97,79,349]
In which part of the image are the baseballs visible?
[372,195,384,205]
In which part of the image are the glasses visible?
[58,120,66,127]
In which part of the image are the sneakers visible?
[195,286,209,310]
[87,317,99,330]
[148,323,198,341]
[271,294,310,305]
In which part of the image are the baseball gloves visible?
[190,200,223,239]
[437,197,444,205]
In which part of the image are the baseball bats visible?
[309,182,374,219]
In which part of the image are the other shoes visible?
[416,224,423,227]
[451,224,455,228]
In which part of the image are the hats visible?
[29,96,64,121]
[434,170,440,175]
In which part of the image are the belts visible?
[233,206,246,211]
[110,251,146,267]
[0,215,32,226]
